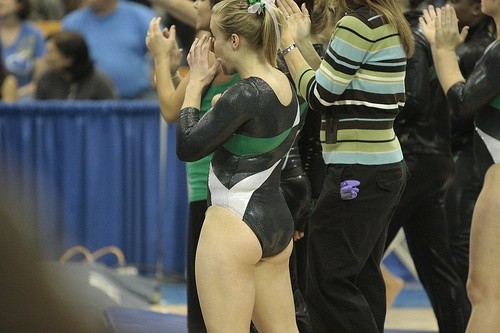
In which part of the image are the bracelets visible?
[276,44,301,56]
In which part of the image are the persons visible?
[262,1,334,212]
[35,30,117,103]
[398,0,500,333]
[177,1,301,333]
[0,0,43,104]
[147,1,258,333]
[369,2,429,299]
[65,1,169,102]
[0,41,21,104]
[267,1,409,333]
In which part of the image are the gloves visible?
[339,180,360,199]
